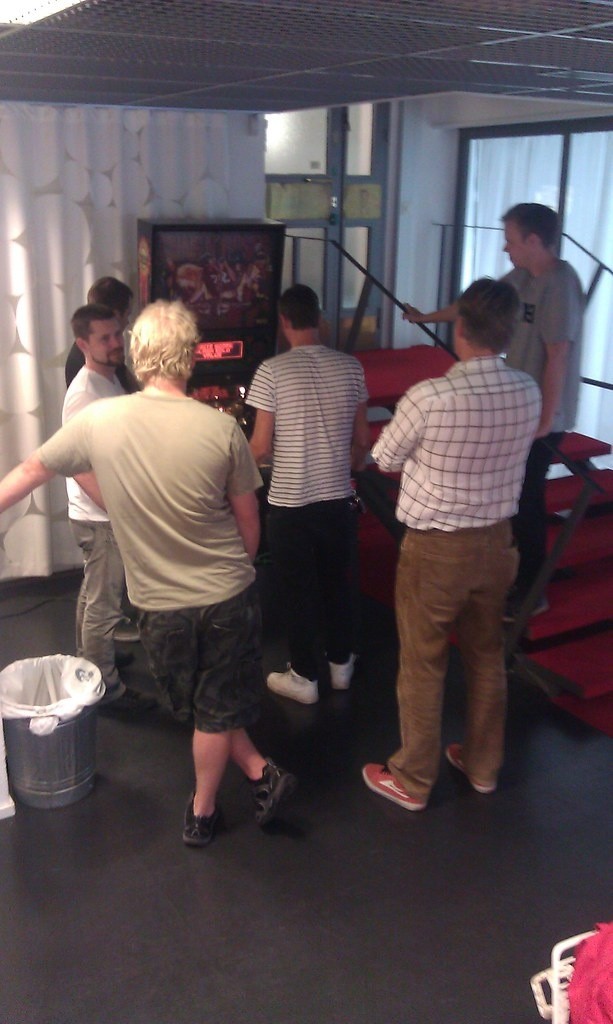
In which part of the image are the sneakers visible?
[181,790,220,844]
[445,744,498,793]
[360,763,427,812]
[266,661,320,706]
[246,757,298,827]
[116,647,134,665]
[99,686,159,710]
[328,652,357,690]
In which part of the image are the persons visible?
[242,282,369,704]
[402,202,583,624]
[362,279,541,810]
[62,304,155,716]
[0,301,296,846]
[65,276,140,392]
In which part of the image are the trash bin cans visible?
[0,654,101,809]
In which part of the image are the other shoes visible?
[503,597,549,622]
